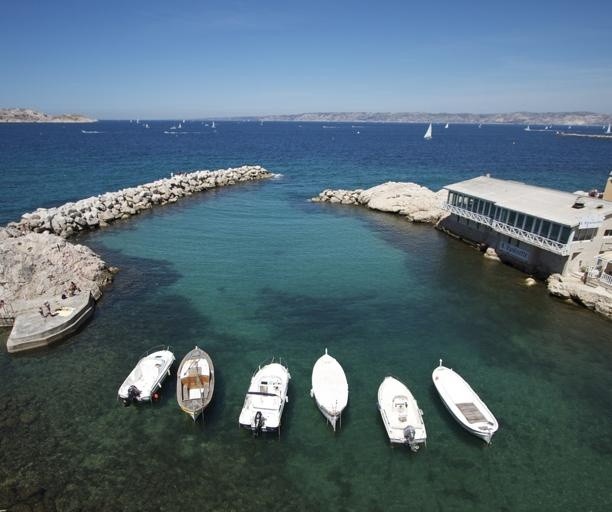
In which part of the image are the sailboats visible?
[423,120,433,140]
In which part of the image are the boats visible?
[176,344,216,423]
[116,343,177,407]
[238,355,291,439]
[376,375,427,453]
[309,347,349,432]
[431,358,499,446]
